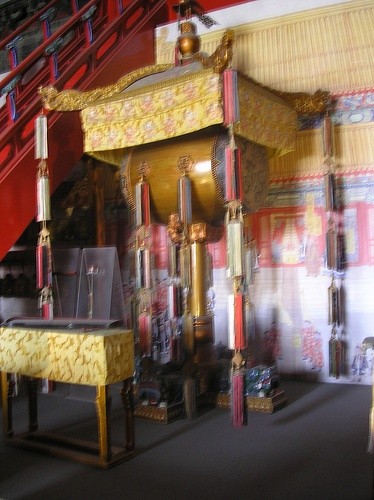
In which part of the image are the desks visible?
[0,326,135,471]
